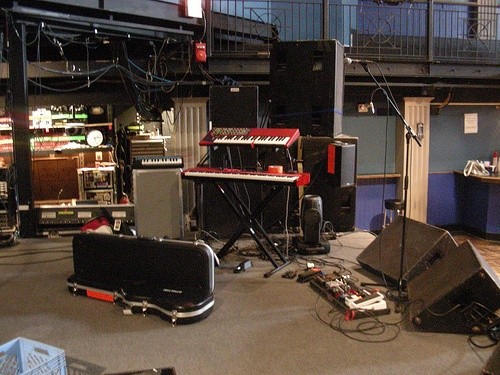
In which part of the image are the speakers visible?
[406,239,500,332]
[272,136,359,233]
[356,215,458,289]
[208,85,259,171]
[269,39,344,136]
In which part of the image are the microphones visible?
[343,57,374,65]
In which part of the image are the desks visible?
[452,169,500,241]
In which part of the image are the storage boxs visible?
[0,337,68,375]
[131,168,185,241]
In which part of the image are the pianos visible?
[133,156,184,169]
[180,167,310,187]
[199,127,300,147]
